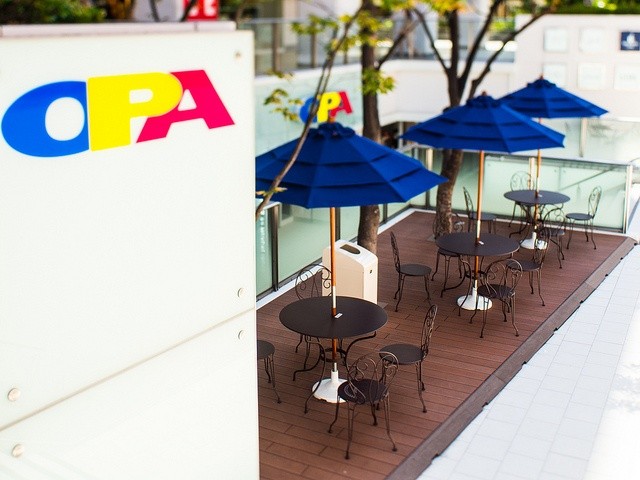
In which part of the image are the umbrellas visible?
[395,90,566,312]
[256,115,451,406]
[495,75,609,251]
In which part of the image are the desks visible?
[279,295,387,414]
[504,189,570,252]
[436,232,520,324]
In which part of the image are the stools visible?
[258,339,281,404]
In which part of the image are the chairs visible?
[534,207,565,269]
[566,186,603,251]
[295,264,344,369]
[431,209,464,290]
[328,350,397,459]
[463,187,496,235]
[469,259,523,338]
[380,304,439,413]
[508,171,541,231]
[390,230,432,311]
[503,226,550,308]
